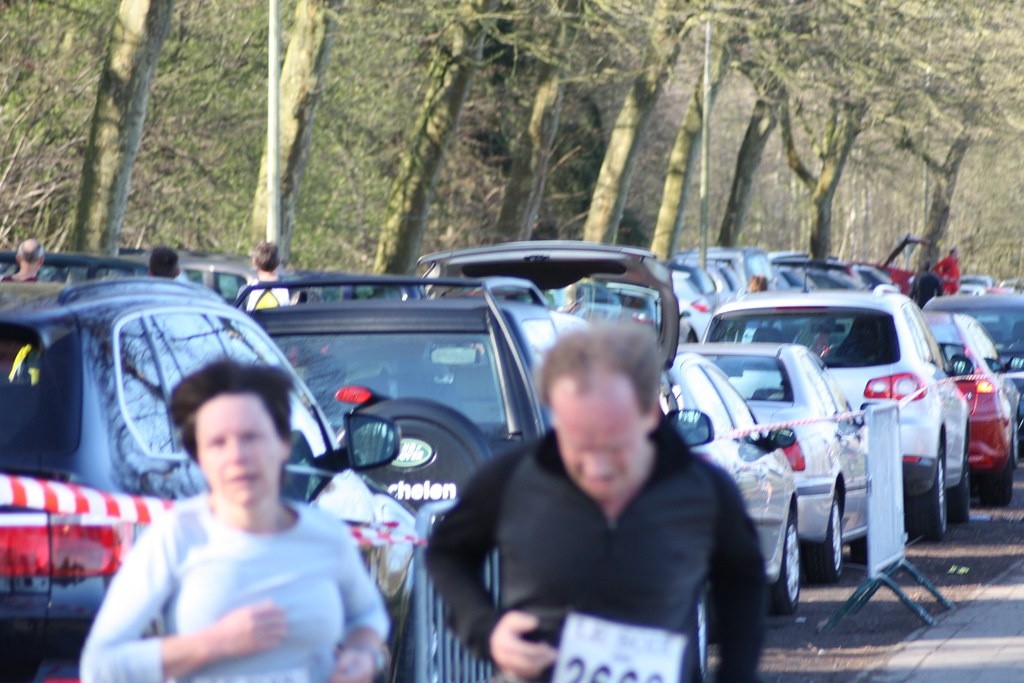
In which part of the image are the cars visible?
[0,239,1024,683]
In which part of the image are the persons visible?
[750,276,767,293]
[909,248,960,310]
[82,361,391,683]
[235,242,290,313]
[423,323,766,683]
[147,247,180,279]
[0,238,45,282]
